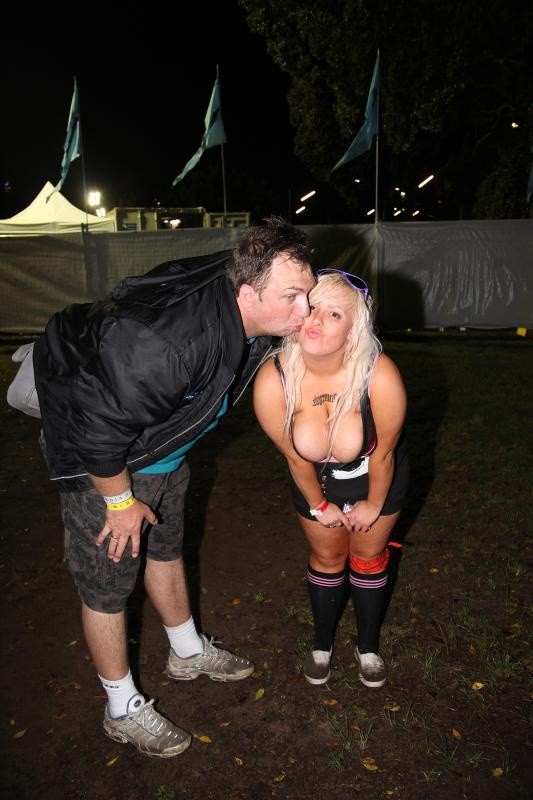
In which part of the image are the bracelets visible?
[310,498,329,516]
[106,495,135,510]
[102,486,133,504]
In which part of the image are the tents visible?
[0,181,115,237]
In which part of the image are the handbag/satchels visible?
[6,342,42,420]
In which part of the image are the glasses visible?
[314,268,368,303]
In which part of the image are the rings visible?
[112,536,119,539]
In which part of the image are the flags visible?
[45,81,83,202]
[171,73,227,189]
[327,54,383,173]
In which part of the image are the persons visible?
[253,263,412,688]
[6,213,316,758]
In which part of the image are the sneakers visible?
[166,633,254,681]
[103,694,191,757]
[303,645,333,685]
[354,646,386,687]
